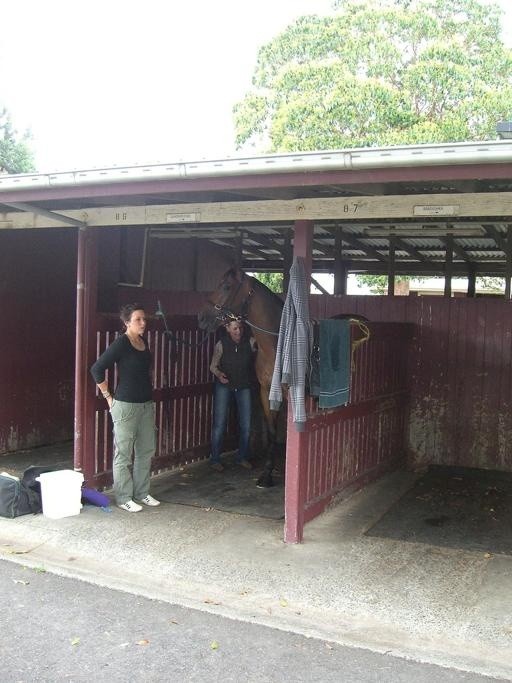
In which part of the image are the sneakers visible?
[116,500,142,512]
[210,463,224,472]
[242,461,253,468]
[133,494,160,507]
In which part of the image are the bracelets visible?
[102,390,111,399]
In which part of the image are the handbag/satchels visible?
[0,465,63,518]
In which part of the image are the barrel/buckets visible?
[35,470,84,520]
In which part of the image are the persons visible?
[208,320,257,472]
[91,301,160,513]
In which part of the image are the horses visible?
[196,266,371,490]
